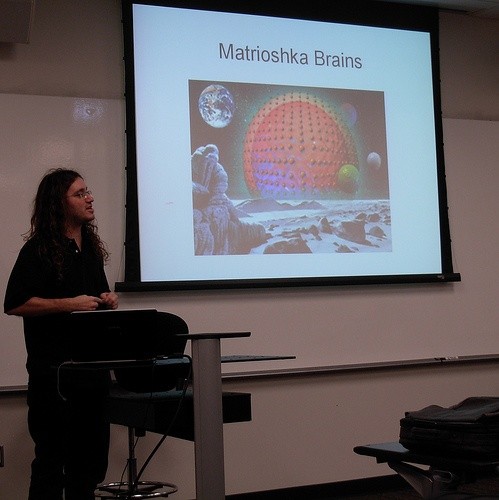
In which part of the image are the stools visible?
[55,309,192,500]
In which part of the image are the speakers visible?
[0,0,35,43]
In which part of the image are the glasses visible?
[63,190,91,199]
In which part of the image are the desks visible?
[353,443,499,500]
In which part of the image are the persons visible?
[3,168,120,500]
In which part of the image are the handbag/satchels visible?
[399,395,499,457]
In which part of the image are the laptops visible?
[70,308,157,366]
